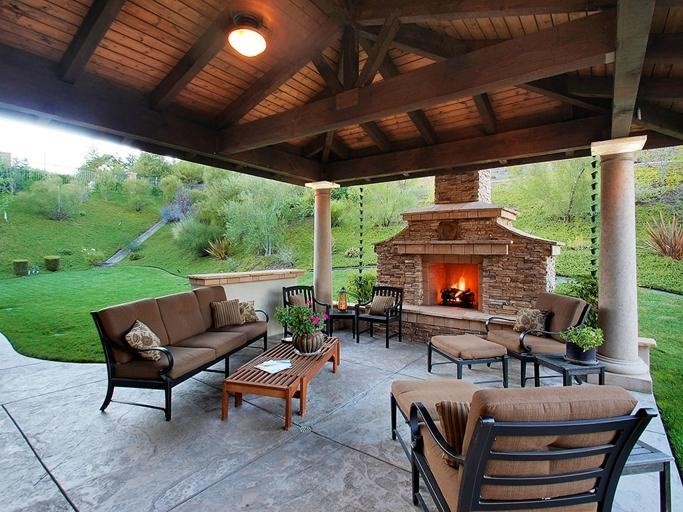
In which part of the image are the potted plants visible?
[557,323,607,365]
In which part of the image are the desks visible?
[532,351,606,387]
[220,335,342,431]
[328,308,356,339]
[619,437,676,512]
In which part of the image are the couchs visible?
[91,283,269,422]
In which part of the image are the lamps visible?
[335,285,349,312]
[226,8,269,58]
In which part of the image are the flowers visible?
[272,300,330,339]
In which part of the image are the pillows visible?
[366,295,394,317]
[237,299,259,324]
[433,398,470,470]
[208,297,243,330]
[289,292,305,306]
[510,307,552,337]
[124,318,163,363]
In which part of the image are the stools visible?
[425,332,510,388]
[389,378,481,465]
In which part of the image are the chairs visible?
[355,285,405,348]
[408,384,659,512]
[281,284,330,338]
[484,291,592,387]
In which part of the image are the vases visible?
[290,327,325,356]
